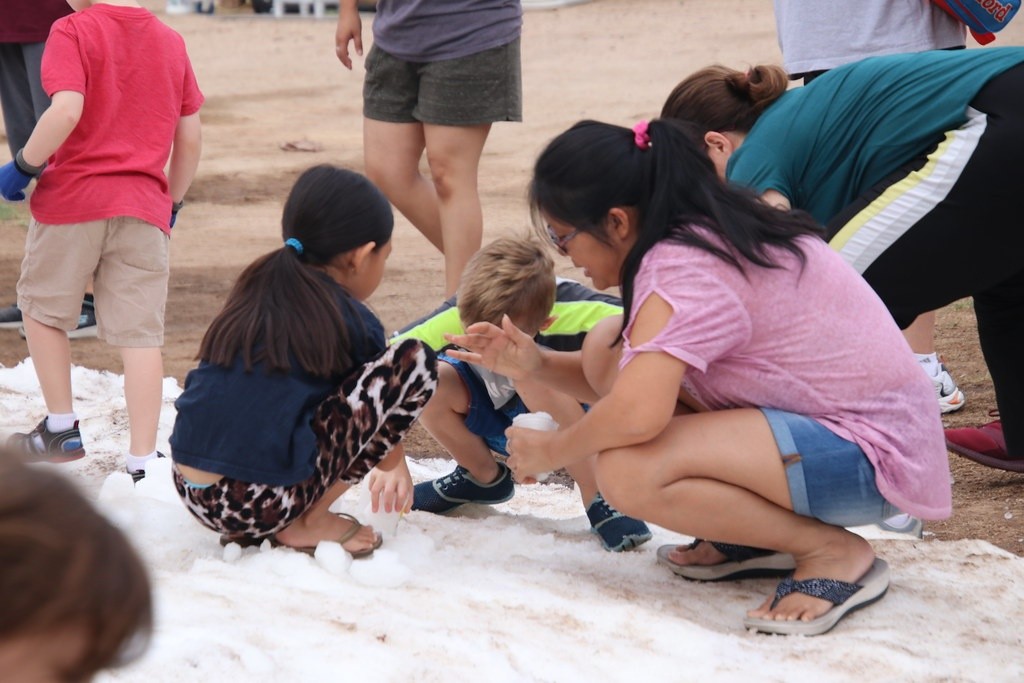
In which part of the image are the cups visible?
[368,487,408,536]
[512,415,558,481]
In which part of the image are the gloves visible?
[0,148,46,201]
[170,200,184,229]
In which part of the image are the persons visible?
[169,163,440,559]
[386,235,652,553]
[0,0,97,338]
[0,439,155,683]
[443,121,952,636]
[774,0,968,415]
[336,0,522,300]
[660,46,1024,472]
[0,0,204,483]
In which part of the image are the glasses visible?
[551,227,582,257]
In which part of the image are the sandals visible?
[0,416,86,463]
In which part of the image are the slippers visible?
[745,557,889,635]
[268,513,384,559]
[658,537,796,583]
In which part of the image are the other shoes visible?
[944,409,1023,472]
[19,294,98,339]
[0,304,23,330]
[931,363,965,414]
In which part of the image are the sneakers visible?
[126,451,166,482]
[412,461,514,515]
[586,493,653,553]
